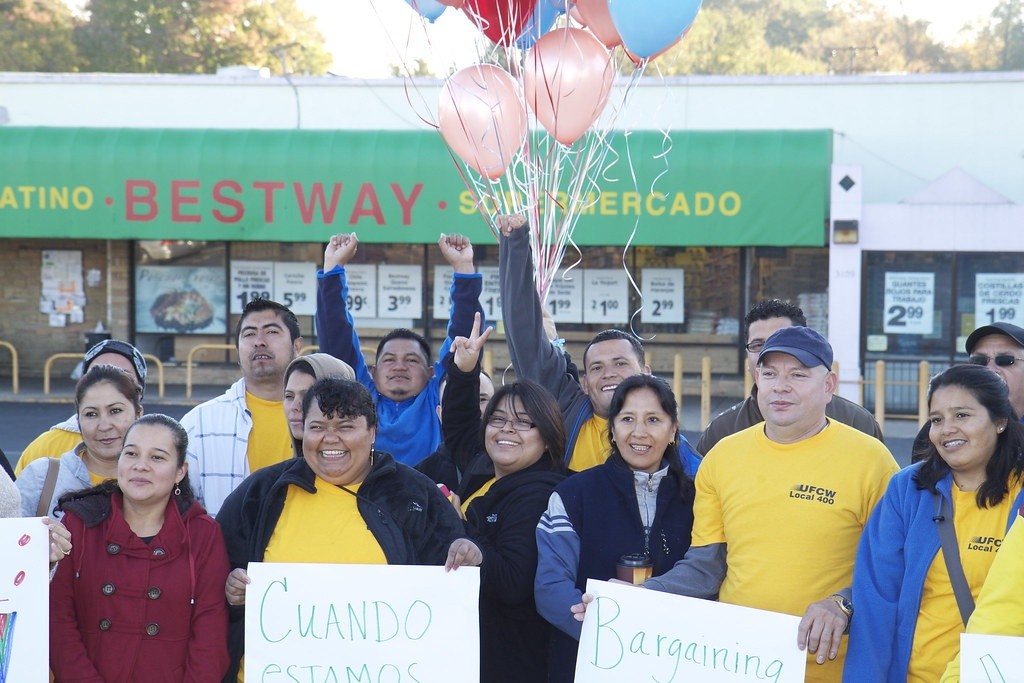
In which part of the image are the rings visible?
[61,549,71,555]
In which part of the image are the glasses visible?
[969,355,1024,366]
[745,342,765,353]
[487,415,536,431]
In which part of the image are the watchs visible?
[827,594,853,634]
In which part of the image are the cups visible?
[437,483,466,520]
[615,554,654,587]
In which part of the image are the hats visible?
[756,326,833,371]
[965,322,1024,354]
[81,339,147,392]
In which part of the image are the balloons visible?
[408,1,701,181]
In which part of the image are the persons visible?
[412,366,495,491]
[842,365,1024,683]
[910,322,1024,465]
[0,449,16,482]
[283,353,355,457]
[177,300,304,519]
[14,340,147,477]
[318,232,484,468]
[572,326,901,683]
[14,364,143,515]
[215,379,484,683]
[435,376,569,683]
[49,413,230,683]
[0,464,72,683]
[498,213,704,482]
[696,299,884,456]
[533,375,695,683]
